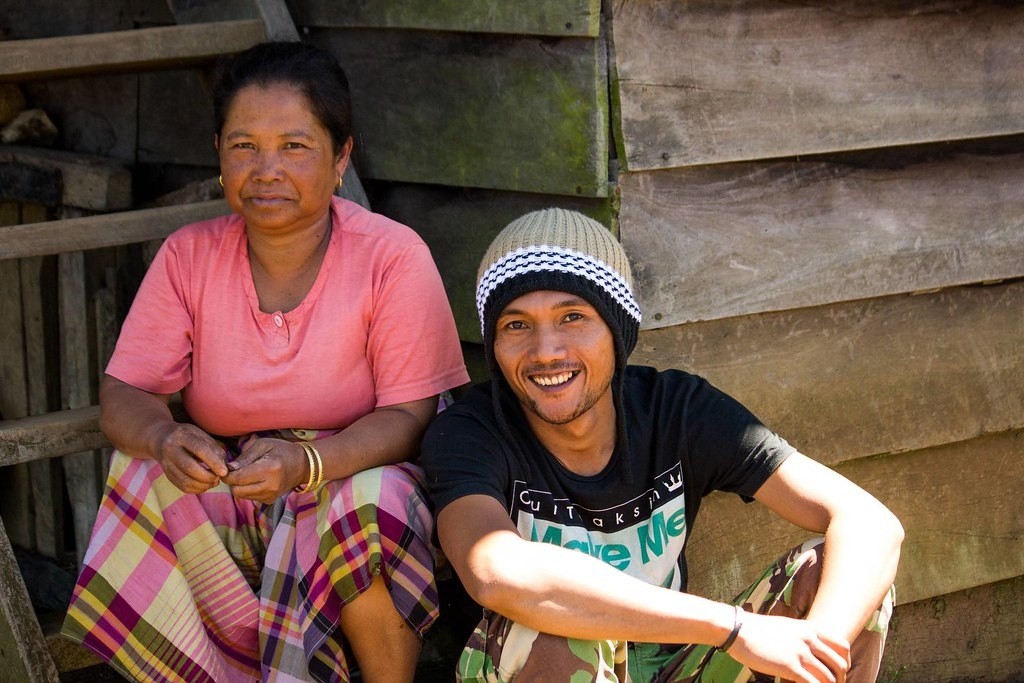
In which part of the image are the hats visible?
[474,208,641,488]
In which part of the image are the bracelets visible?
[292,442,324,494]
[716,605,744,653]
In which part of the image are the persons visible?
[421,208,905,683]
[60,42,471,683]
